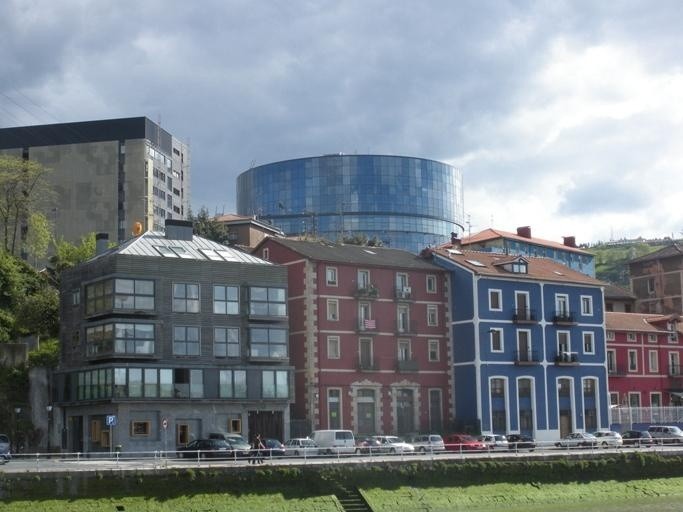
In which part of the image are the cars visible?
[554,426,683,449]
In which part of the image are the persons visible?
[253,433,265,464]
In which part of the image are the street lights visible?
[15,406,21,454]
[46,405,53,458]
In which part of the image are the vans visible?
[0,433,12,465]
